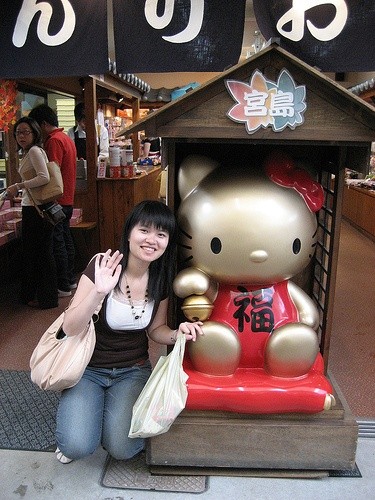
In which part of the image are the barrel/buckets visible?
[121,166,133,178]
[109,146,120,166]
[120,150,133,166]
[110,166,121,178]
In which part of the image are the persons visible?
[53,199,205,465]
[5,116,66,308]
[26,104,78,297]
[64,103,111,163]
[143,132,162,159]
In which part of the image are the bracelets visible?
[171,330,177,344]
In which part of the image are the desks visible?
[329,179,375,244]
[70,222,97,231]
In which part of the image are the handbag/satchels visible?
[41,200,66,226]
[21,147,64,205]
[28,309,96,391]
[128,333,189,439]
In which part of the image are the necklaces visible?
[123,272,149,320]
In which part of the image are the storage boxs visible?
[0,195,23,246]
[70,207,84,226]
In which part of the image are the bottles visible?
[97,152,110,178]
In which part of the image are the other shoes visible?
[57,288,72,298]
[54,447,72,463]
[70,283,78,289]
[27,297,58,310]
